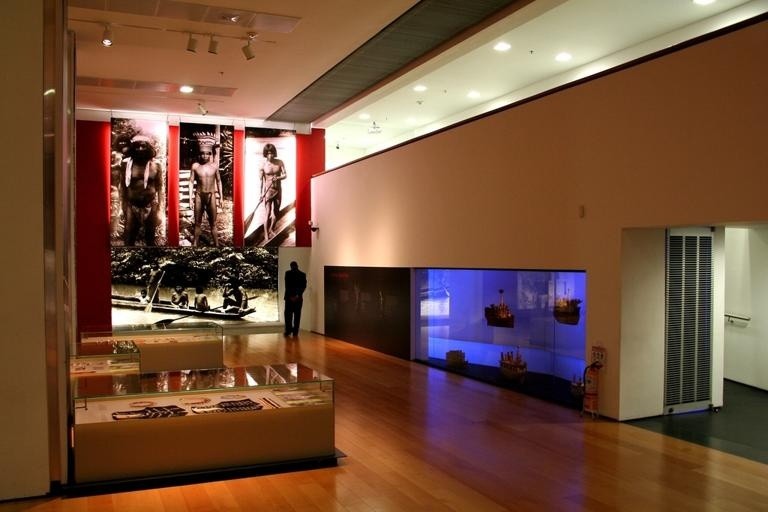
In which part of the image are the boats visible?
[244,201,297,248]
[112,293,257,320]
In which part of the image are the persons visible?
[120,135,164,248]
[124,289,150,303]
[258,144,287,242]
[283,333,301,363]
[281,260,308,338]
[188,144,225,248]
[194,286,211,311]
[146,260,163,304]
[170,283,191,310]
[222,276,248,315]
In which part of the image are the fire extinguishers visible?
[582,361,603,413]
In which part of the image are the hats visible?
[193,131,217,153]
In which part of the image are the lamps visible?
[185,32,255,61]
[98,21,120,49]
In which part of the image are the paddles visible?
[155,296,258,324]
[143,270,165,313]
[243,182,274,235]
